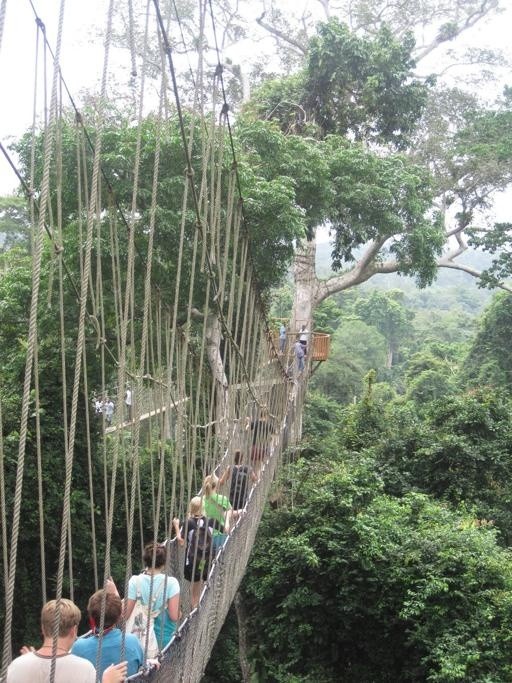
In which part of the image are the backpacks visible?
[189,518,212,568]
[126,576,158,659]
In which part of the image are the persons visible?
[218,450,258,510]
[201,474,246,556]
[103,395,114,426]
[124,385,133,421]
[106,541,182,651]
[6,595,128,682]
[298,324,310,355]
[18,587,161,682]
[95,395,103,416]
[279,322,289,353]
[173,495,232,611]
[289,339,309,370]
[243,407,279,464]
